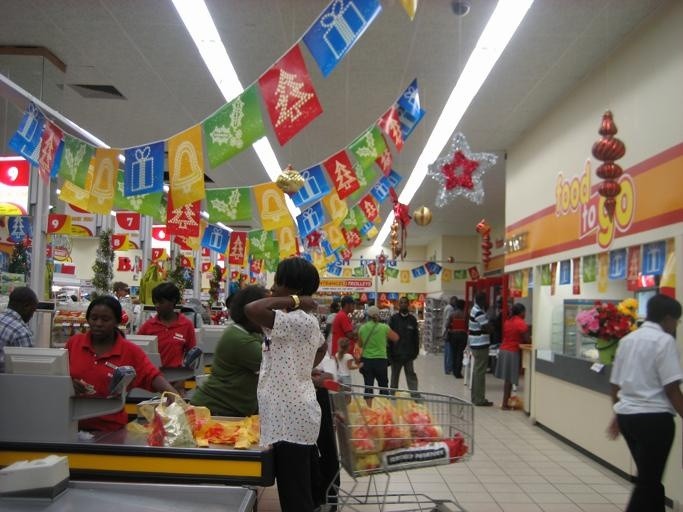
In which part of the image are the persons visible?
[325,302,340,359]
[468,291,495,406]
[0,285,39,359]
[113,281,132,299]
[335,337,358,390]
[190,284,269,416]
[135,282,197,368]
[244,257,325,511]
[330,296,358,362]
[441,296,458,375]
[445,299,468,378]
[64,294,179,401]
[358,305,399,399]
[387,297,420,402]
[493,303,528,409]
[604,294,683,512]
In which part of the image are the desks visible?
[519,344,531,416]
[530,344,683,512]
[125,374,201,412]
[0,414,277,487]
[1,481,255,512]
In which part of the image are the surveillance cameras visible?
[451,0,471,16]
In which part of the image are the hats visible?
[341,296,358,305]
[368,305,380,318]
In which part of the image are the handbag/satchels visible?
[442,328,449,341]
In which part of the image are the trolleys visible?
[315,375,474,512]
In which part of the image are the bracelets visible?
[290,293,300,309]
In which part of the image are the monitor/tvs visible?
[127,335,158,355]
[2,347,75,397]
[199,325,226,353]
